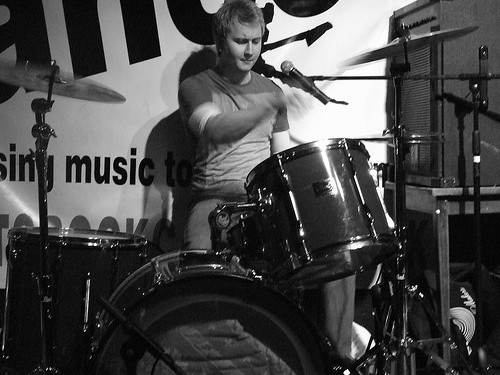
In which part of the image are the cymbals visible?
[359,125,440,144]
[0,64,127,104]
[350,25,480,66]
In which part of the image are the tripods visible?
[348,60,460,375]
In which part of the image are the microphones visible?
[282,61,330,106]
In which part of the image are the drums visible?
[245,138,400,288]
[88,249,352,375]
[207,203,266,270]
[0,227,149,375]
